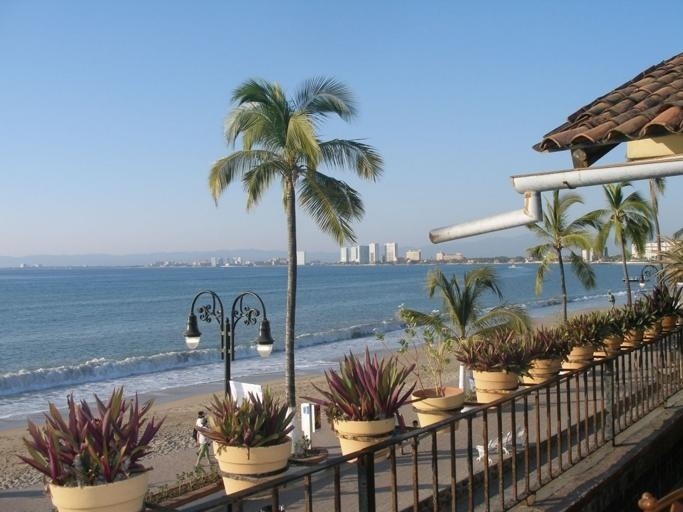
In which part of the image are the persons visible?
[193,411,216,472]
[607,291,615,309]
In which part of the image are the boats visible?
[622,276,649,282]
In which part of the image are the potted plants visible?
[13,383,167,512]
[526,283,682,383]
[194,381,297,501]
[299,345,417,465]
[372,267,531,435]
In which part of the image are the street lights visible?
[182,290,274,400]
[638,265,659,291]
